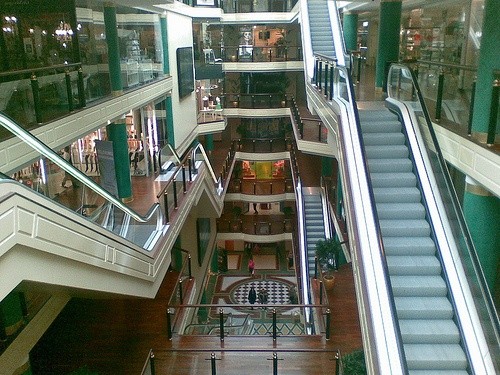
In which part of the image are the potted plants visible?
[316,235,343,291]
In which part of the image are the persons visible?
[61,146,79,190]
[252,202,259,215]
[248,259,255,278]
[259,289,268,310]
[248,287,257,310]
